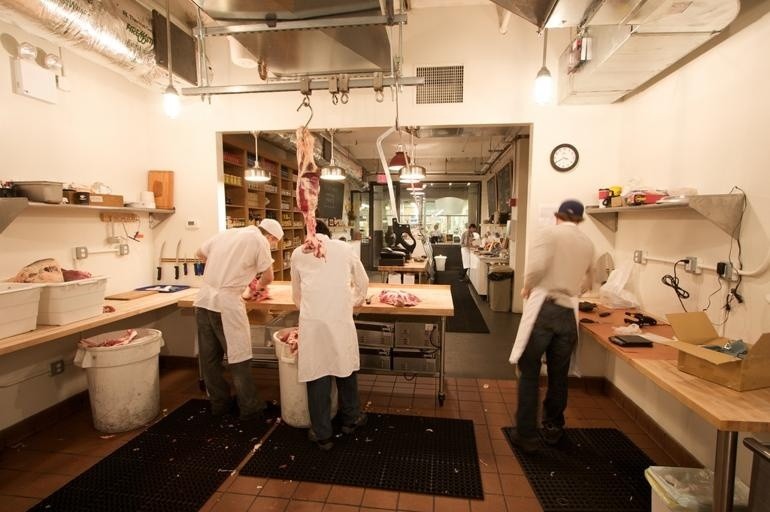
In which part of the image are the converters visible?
[716,263,726,275]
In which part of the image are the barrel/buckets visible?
[403,273,415,285]
[389,272,401,285]
[273,327,339,427]
[74,327,164,435]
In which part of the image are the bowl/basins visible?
[10,180,65,204]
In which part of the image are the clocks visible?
[549,143,581,172]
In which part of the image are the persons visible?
[192,216,284,424]
[458,223,476,282]
[433,225,443,236]
[291,219,369,452]
[507,199,596,455]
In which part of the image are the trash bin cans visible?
[644,465,750,512]
[489,265,513,312]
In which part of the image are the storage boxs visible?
[36,269,110,327]
[639,310,769,394]
[0,281,44,341]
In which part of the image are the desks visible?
[0,283,201,433]
[377,255,429,284]
[575,299,768,508]
[175,278,458,409]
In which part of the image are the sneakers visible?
[339,411,371,433]
[541,423,567,446]
[305,425,338,451]
[508,425,543,457]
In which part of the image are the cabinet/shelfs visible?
[221,135,307,282]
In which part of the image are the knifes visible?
[173,239,182,284]
[193,249,198,276]
[182,252,188,276]
[157,240,165,280]
[197,259,200,275]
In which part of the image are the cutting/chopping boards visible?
[104,289,159,301]
[148,171,174,210]
[177,280,455,317]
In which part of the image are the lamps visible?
[40,50,60,103]
[160,4,181,117]
[530,26,558,107]
[397,126,428,183]
[319,132,349,182]
[15,42,39,97]
[386,143,411,171]
[243,132,271,184]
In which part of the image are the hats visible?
[257,218,284,240]
[554,200,585,220]
